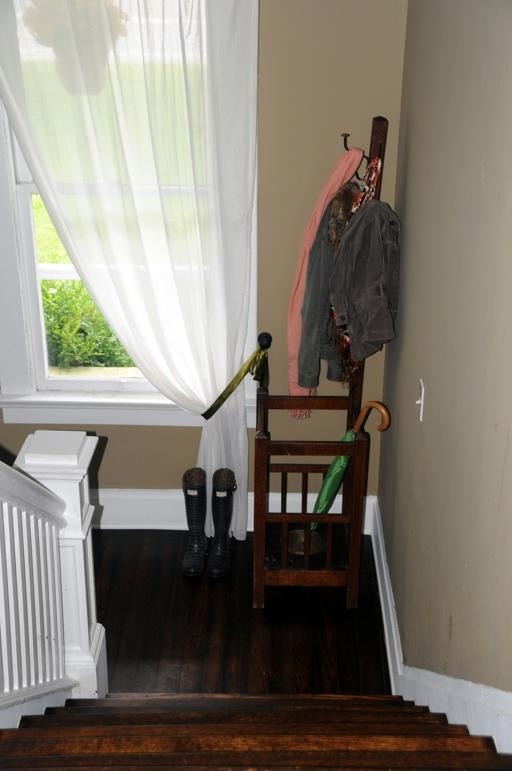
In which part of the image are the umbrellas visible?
[305,401,390,539]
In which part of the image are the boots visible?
[183,468,207,576]
[210,467,233,577]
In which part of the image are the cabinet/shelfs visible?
[251,333,369,609]
